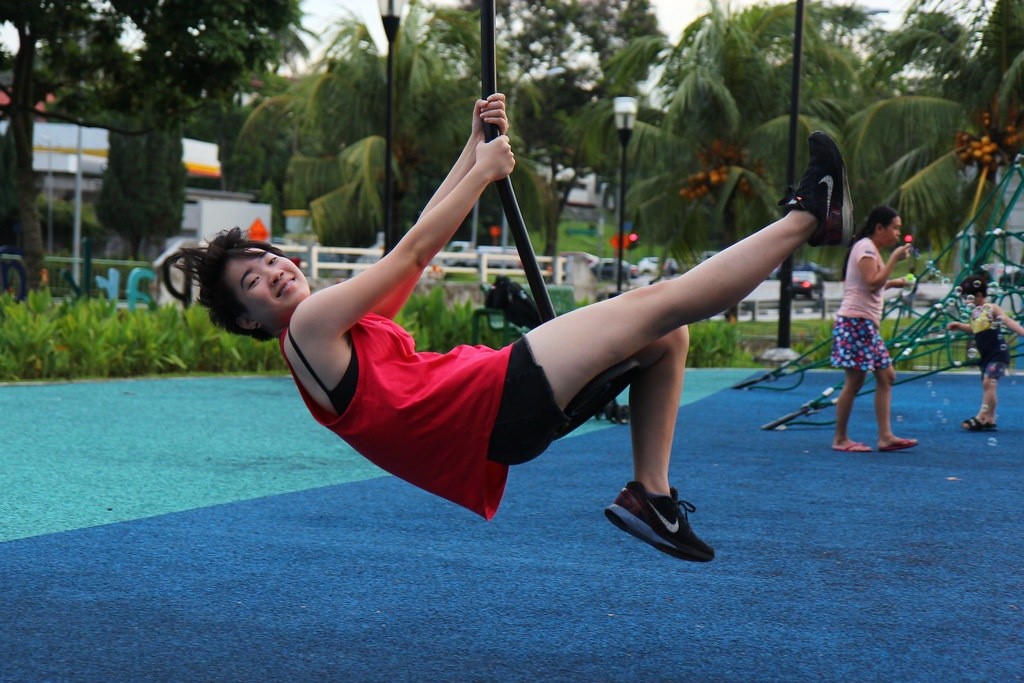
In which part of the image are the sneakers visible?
[605,481,714,563]
[788,130,853,247]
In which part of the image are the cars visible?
[791,270,824,300]
[640,256,676,275]
[587,257,640,282]
[982,263,1024,284]
[771,260,843,282]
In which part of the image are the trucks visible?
[445,240,523,273]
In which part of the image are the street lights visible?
[74,99,88,293]
[614,92,640,295]
[381,0,409,261]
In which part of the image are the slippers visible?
[833,443,872,452]
[879,439,919,451]
[962,416,997,430]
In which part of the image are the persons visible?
[831,206,918,452]
[161,93,853,563]
[946,276,1024,432]
